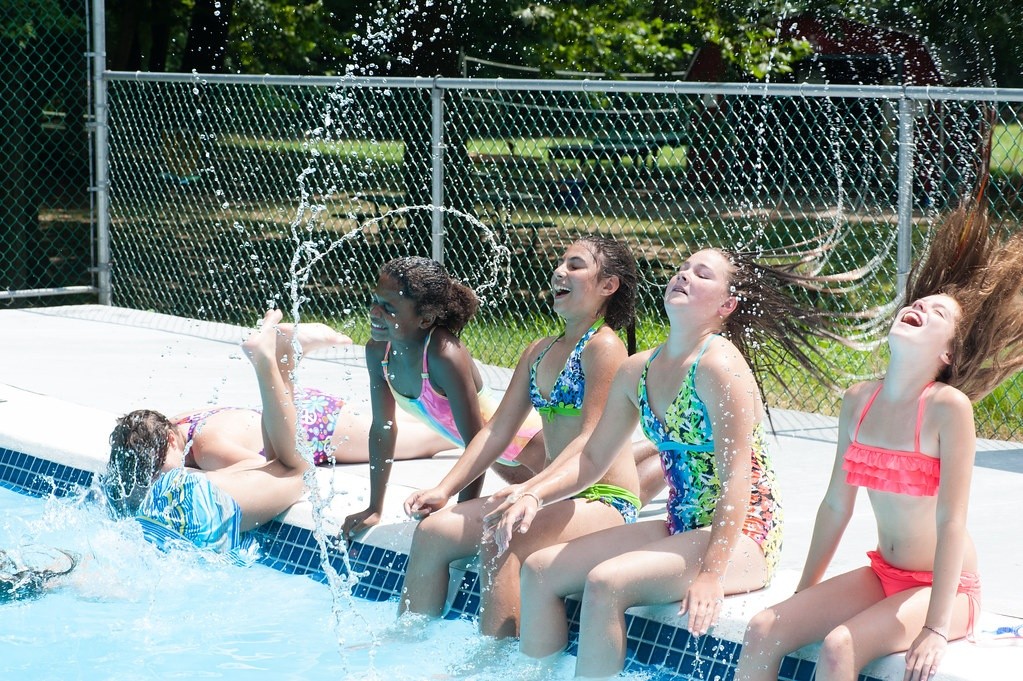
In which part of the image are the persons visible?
[733,110,1023,681]
[342,253,666,541]
[0,308,352,605]
[399,233,642,639]
[495,222,904,675]
[108,388,456,506]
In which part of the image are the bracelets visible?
[519,492,542,511]
[923,626,948,640]
